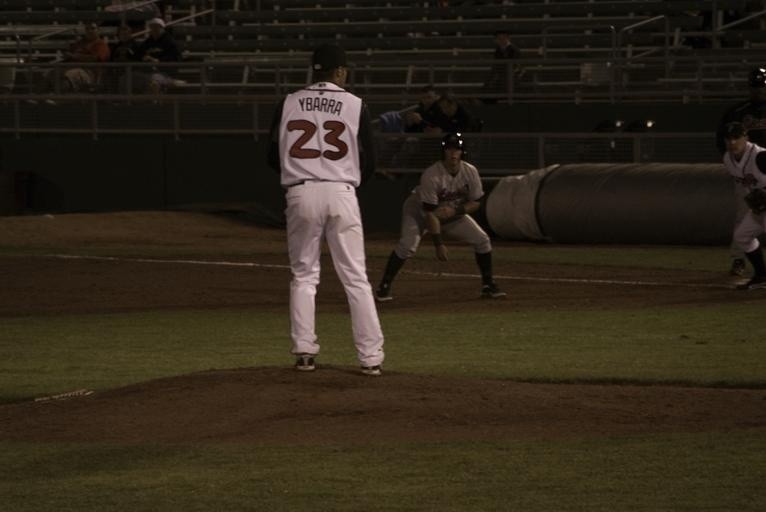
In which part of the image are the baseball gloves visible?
[743,186,766,216]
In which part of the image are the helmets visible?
[438,133,464,151]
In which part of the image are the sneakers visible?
[730,258,745,276]
[480,285,507,300]
[737,277,765,292]
[296,353,316,370]
[376,284,392,303]
[364,363,383,377]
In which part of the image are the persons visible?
[718,122,766,290]
[571,24,599,82]
[268,45,385,377]
[54,17,203,94]
[376,131,506,301]
[716,67,766,274]
[372,29,521,133]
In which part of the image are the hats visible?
[147,18,165,29]
[720,124,747,141]
[312,43,349,70]
